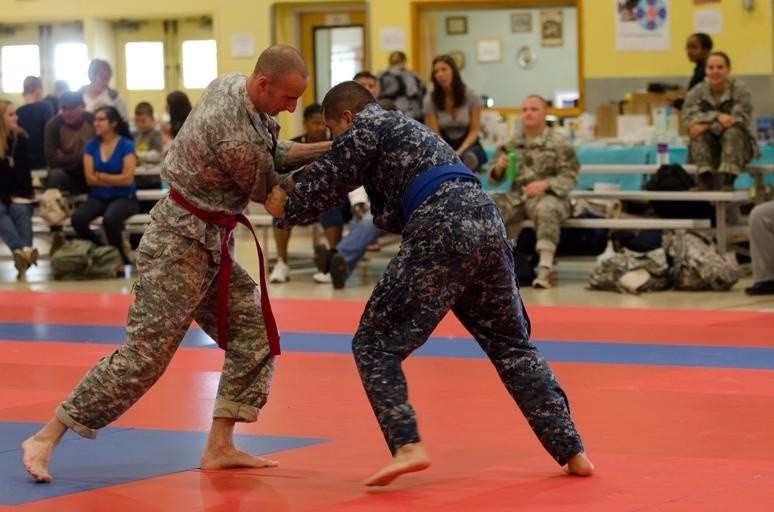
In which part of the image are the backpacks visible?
[34,187,75,224]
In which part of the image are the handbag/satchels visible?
[584,239,667,295]
[50,239,122,281]
[662,227,745,291]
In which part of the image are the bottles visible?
[503,149,519,180]
[656,132,671,169]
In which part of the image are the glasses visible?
[93,117,105,122]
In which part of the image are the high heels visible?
[14,249,27,279]
[24,246,39,265]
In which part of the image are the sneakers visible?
[532,267,552,288]
[330,255,347,289]
[314,243,337,274]
[269,256,289,282]
[746,280,774,295]
[312,272,333,282]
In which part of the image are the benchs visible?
[519,163,773,276]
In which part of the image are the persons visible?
[488,94,581,289]
[0,58,192,278]
[266,82,597,487]
[268,51,487,287]
[21,44,334,482]
[682,33,774,294]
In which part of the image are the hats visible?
[57,91,83,108]
